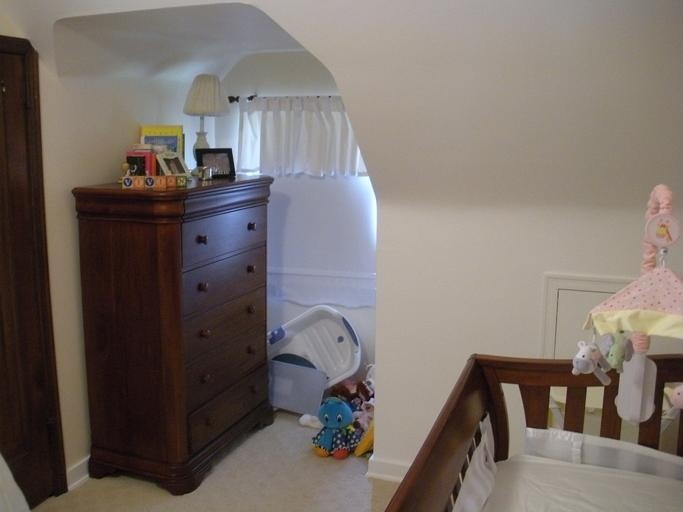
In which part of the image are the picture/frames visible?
[194,147,236,178]
[155,151,191,178]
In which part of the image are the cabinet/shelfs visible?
[72,175,276,496]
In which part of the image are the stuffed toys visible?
[298,364,375,460]
[660,385,682,430]
[571,328,633,386]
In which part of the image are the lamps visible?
[182,74,229,177]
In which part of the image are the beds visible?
[384,352,683,512]
